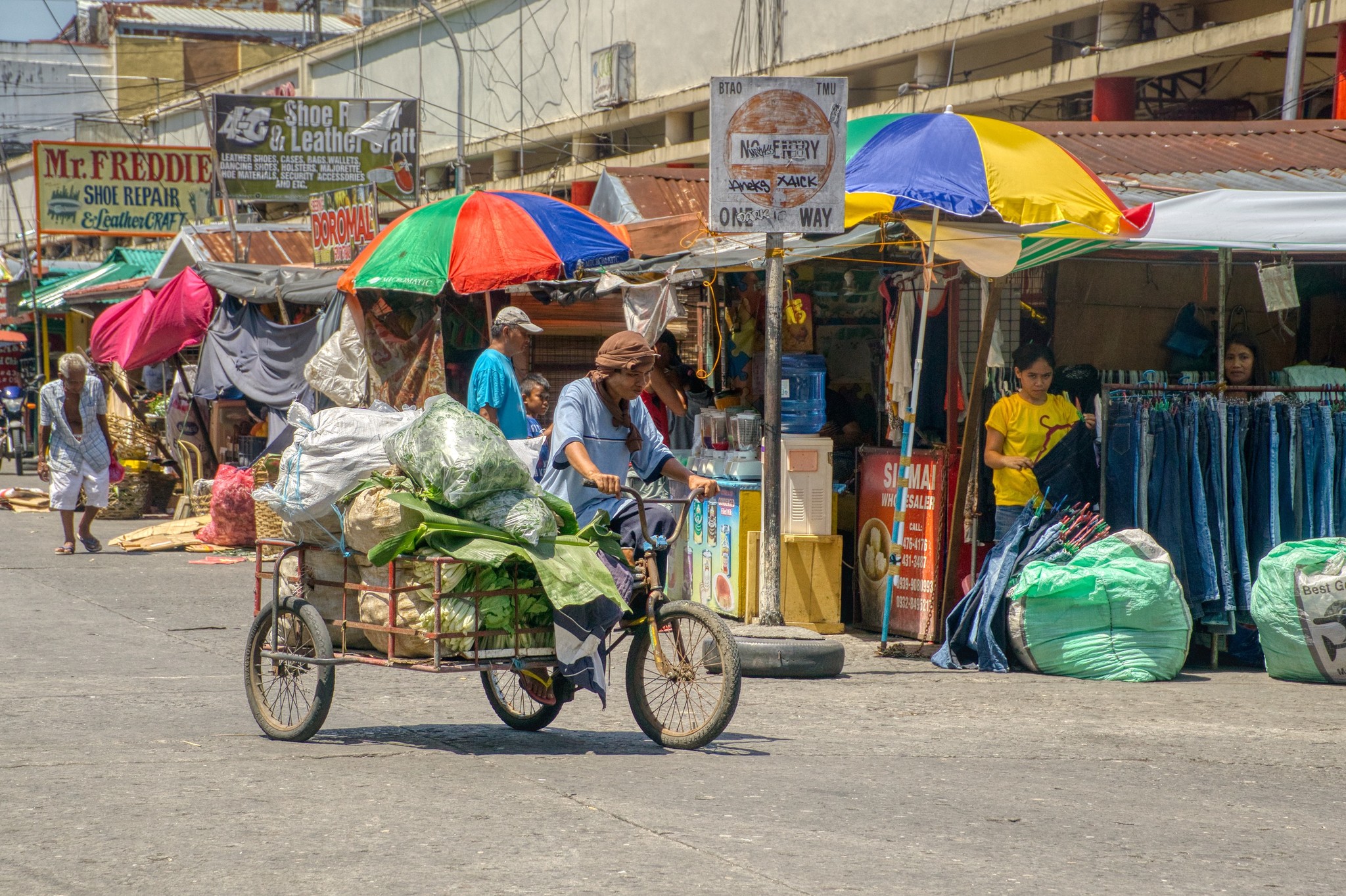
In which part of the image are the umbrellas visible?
[336,185,632,347]
[1020,188,1345,253]
[845,105,1156,584]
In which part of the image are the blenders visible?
[686,406,762,481]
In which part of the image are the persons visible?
[511,331,721,705]
[984,344,1096,546]
[466,306,544,440]
[519,373,553,483]
[140,359,174,395]
[1224,332,1288,400]
[623,328,705,515]
[37,353,118,556]
[812,372,875,494]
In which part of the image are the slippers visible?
[516,669,556,707]
[76,533,103,552]
[613,614,673,633]
[55,545,76,555]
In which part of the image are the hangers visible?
[1108,371,1346,412]
[1029,485,1111,555]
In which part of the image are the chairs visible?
[173,440,203,521]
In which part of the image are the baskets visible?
[253,463,286,556]
[189,494,212,517]
[78,414,162,520]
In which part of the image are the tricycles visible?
[243,470,744,749]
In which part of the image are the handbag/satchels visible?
[382,393,558,546]
[109,441,126,486]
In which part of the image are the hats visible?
[493,306,544,333]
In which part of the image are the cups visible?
[858,518,891,626]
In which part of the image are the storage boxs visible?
[237,435,266,467]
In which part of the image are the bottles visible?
[780,354,828,435]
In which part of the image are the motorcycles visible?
[0,373,49,475]
